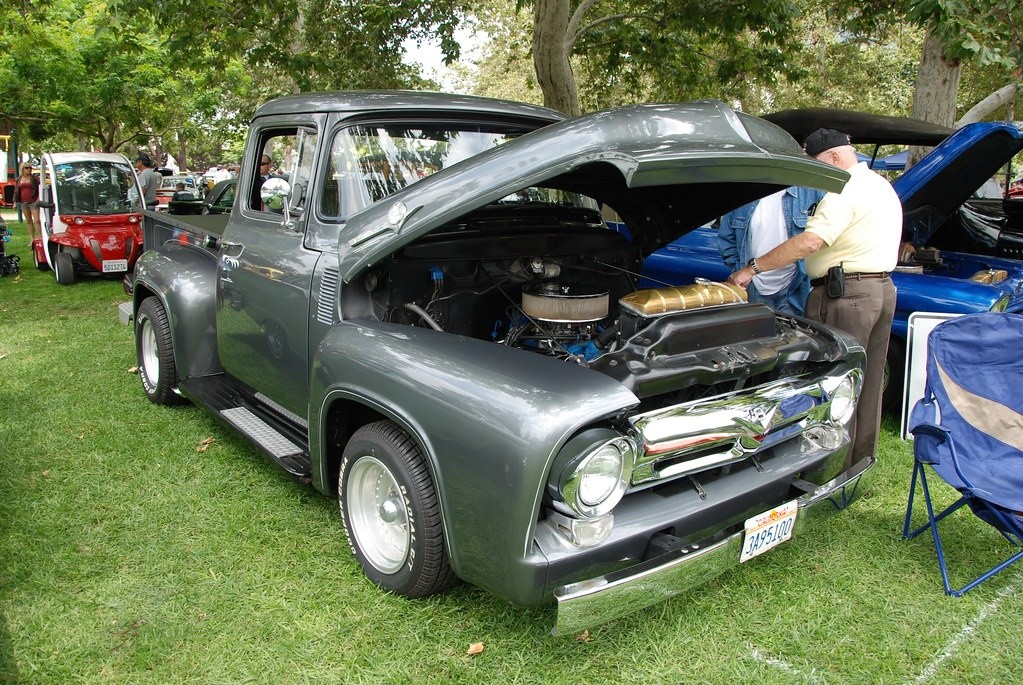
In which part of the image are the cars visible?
[0,136,585,209]
[934,196,1023,261]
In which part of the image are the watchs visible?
[748,258,760,274]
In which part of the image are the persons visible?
[153,167,162,190]
[214,164,232,186]
[130,154,156,207]
[13,162,42,246]
[257,155,275,186]
[974,173,1003,199]
[718,130,903,472]
[172,183,194,201]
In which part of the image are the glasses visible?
[24,167,32,170]
[261,161,270,167]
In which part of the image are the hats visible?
[137,155,150,161]
[803,127,851,158]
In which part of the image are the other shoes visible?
[28,240,32,247]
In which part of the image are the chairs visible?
[903,315,1023,595]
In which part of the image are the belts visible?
[811,272,893,288]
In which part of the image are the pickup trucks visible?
[120,89,877,638]
[605,118,1022,409]
